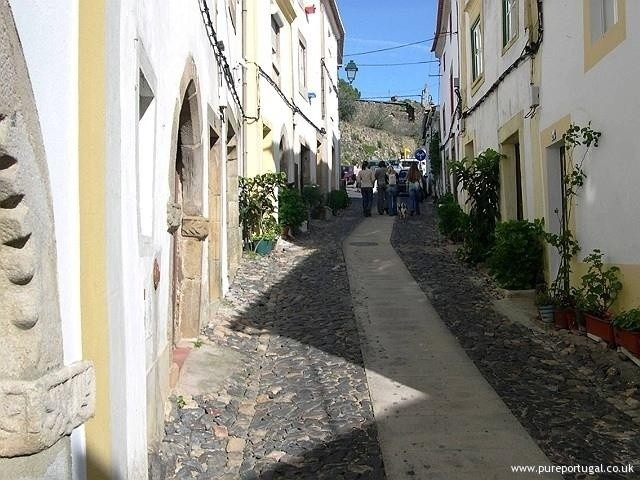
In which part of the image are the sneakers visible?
[364,209,396,217]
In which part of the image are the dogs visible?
[397,203,408,220]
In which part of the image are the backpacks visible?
[387,173,396,185]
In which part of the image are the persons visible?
[405,162,423,217]
[353,162,362,188]
[357,160,375,217]
[375,160,389,214]
[385,165,399,217]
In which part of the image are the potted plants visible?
[236,170,288,254]
[570,246,640,357]
[277,185,310,237]
[535,119,602,329]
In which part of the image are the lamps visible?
[345,60,358,84]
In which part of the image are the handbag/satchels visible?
[416,188,426,201]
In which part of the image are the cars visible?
[341,157,426,199]
